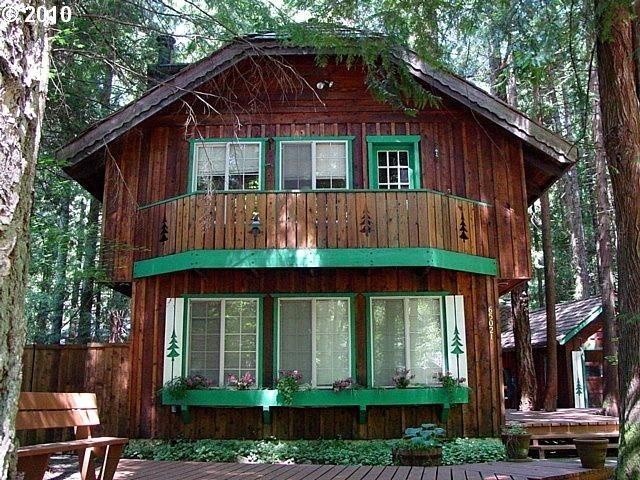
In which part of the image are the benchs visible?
[10,390,130,480]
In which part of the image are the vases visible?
[572,435,610,469]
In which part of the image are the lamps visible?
[315,79,325,90]
[323,79,334,88]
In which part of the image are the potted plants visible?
[395,423,444,465]
[501,421,533,459]
